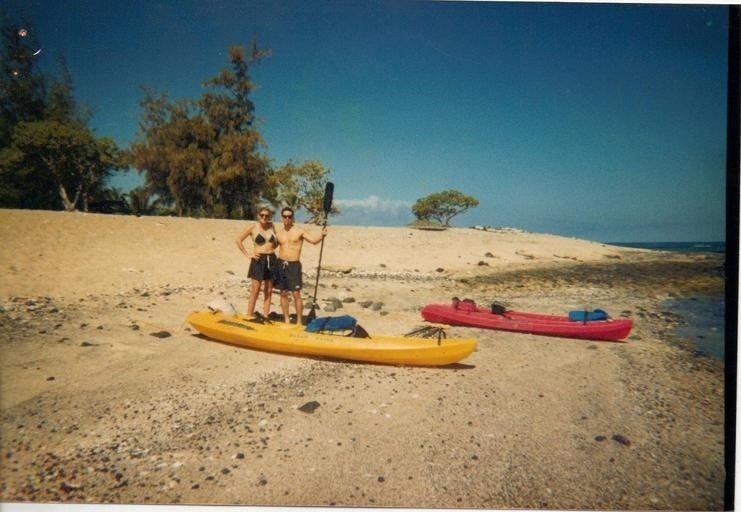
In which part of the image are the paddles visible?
[304,182,334,326]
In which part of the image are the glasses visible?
[282,215,293,218]
[258,214,269,217]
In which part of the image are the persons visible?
[237,205,278,320]
[274,208,328,326]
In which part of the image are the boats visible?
[421,298,634,341]
[184,310,477,365]
